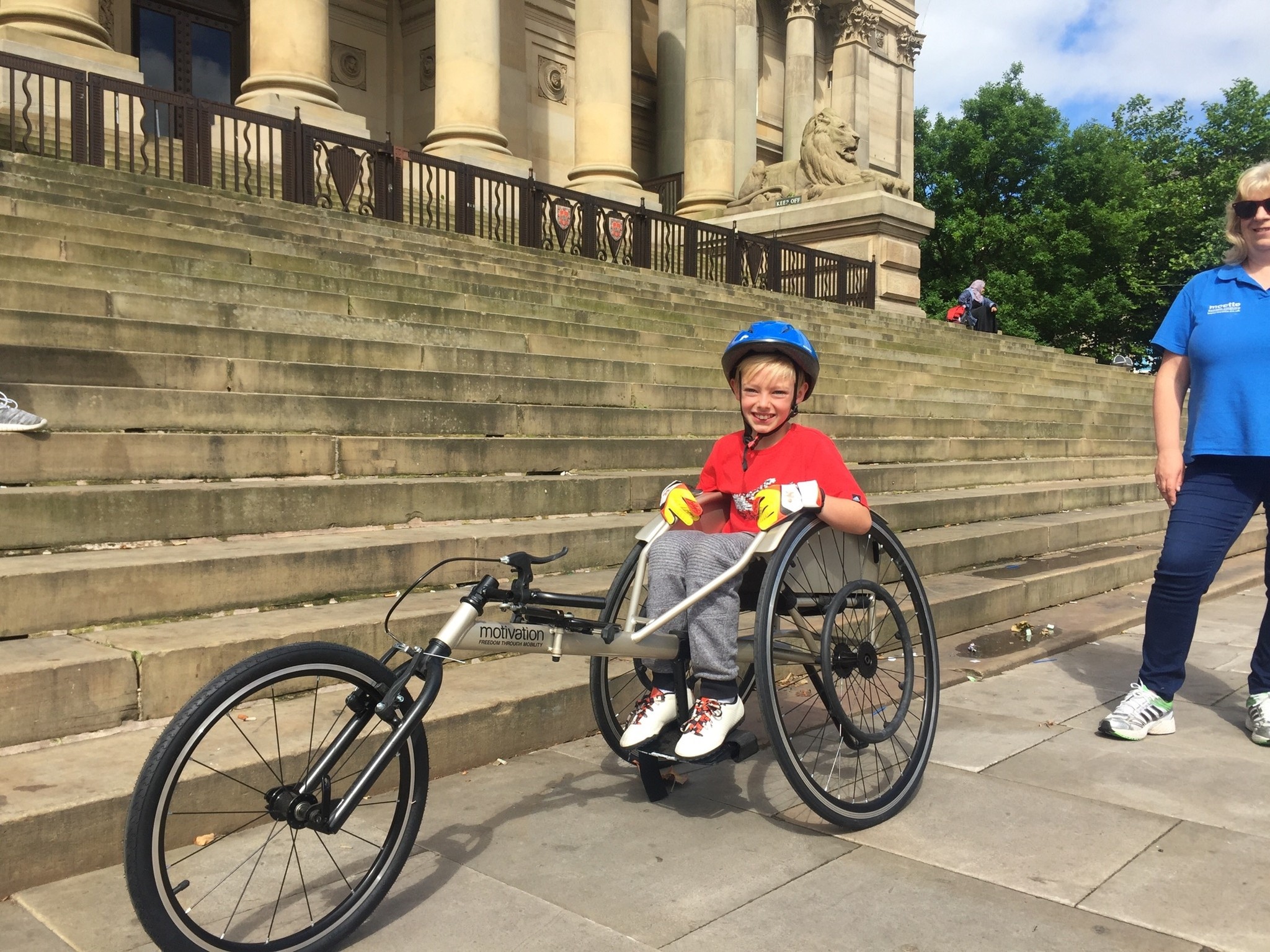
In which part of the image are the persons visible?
[958,279,1000,333]
[0,391,48,432]
[617,323,872,758]
[1099,164,1269,746]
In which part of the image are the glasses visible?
[1232,197,1270,219]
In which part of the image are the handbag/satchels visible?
[947,305,969,324]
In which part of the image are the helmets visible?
[721,320,820,402]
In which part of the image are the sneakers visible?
[620,687,697,750]
[1245,691,1270,744]
[0,392,48,431]
[1098,677,1176,741]
[674,692,745,761]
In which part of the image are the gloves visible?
[752,479,826,532]
[660,480,704,526]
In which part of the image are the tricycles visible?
[124,491,942,952]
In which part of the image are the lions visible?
[726,108,910,208]
